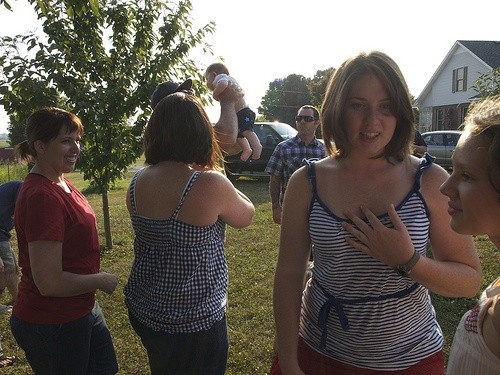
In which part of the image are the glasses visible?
[294,115,316,123]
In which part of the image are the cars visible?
[415,127,474,171]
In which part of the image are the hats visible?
[150,79,193,110]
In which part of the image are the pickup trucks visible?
[218,120,336,184]
